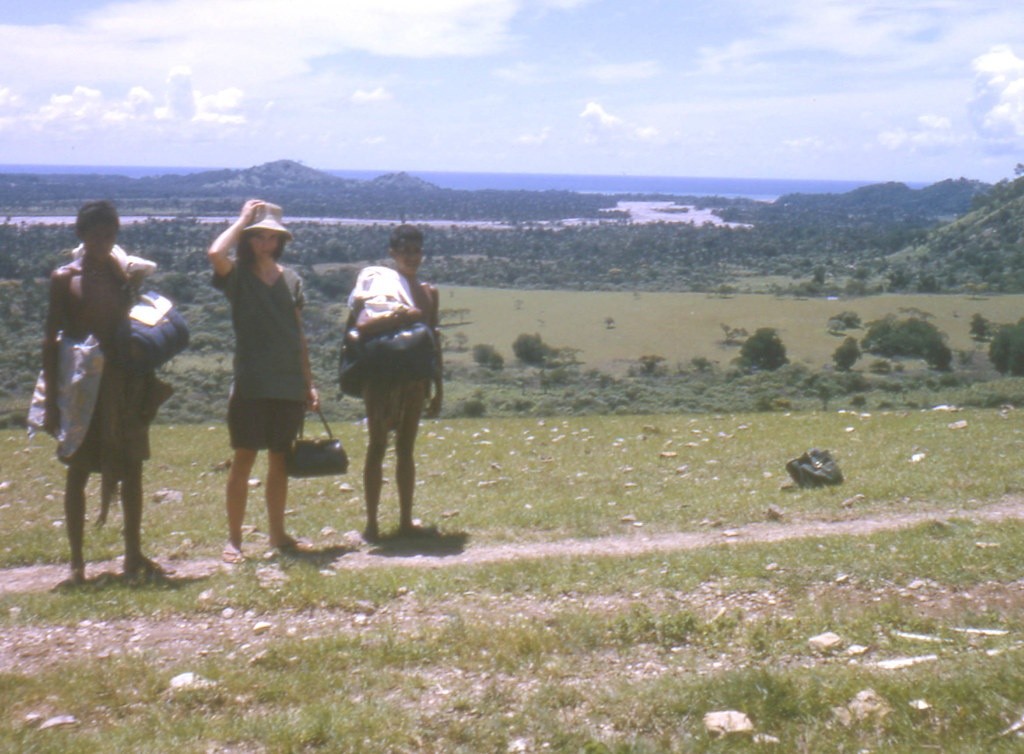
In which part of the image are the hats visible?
[242,203,292,238]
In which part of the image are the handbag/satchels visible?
[291,405,348,478]
[785,449,842,486]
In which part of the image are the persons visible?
[207,199,319,563]
[43,201,163,593]
[340,224,443,545]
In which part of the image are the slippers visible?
[269,540,312,554]
[224,543,242,563]
[130,558,176,575]
